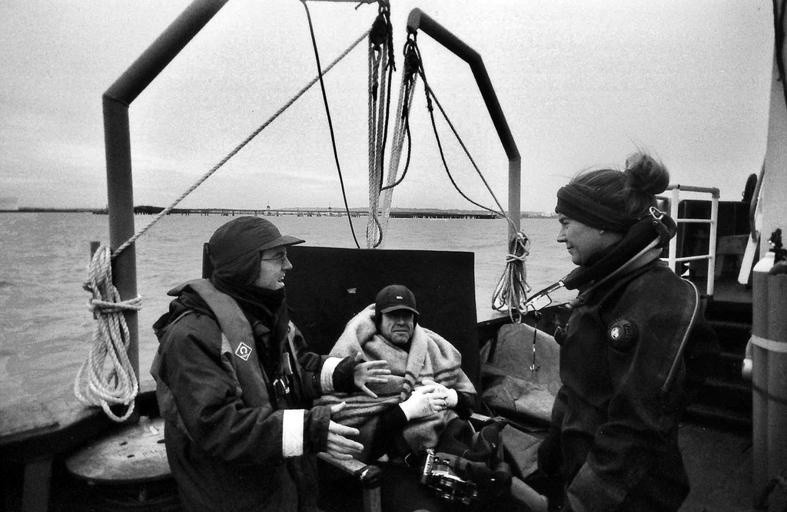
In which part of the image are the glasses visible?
[260,251,286,266]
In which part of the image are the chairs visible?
[316,413,508,512]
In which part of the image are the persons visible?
[531,149,702,511]
[320,280,549,511]
[148,215,390,510]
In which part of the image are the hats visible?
[374,283,421,319]
[204,214,309,270]
